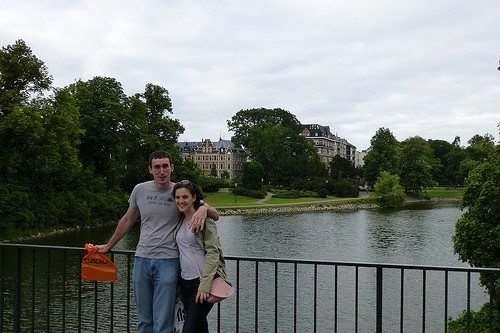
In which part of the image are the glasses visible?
[174,180,192,188]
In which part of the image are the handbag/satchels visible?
[173,297,185,333]
[197,277,235,303]
[81,243,118,282]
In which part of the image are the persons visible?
[172,180,226,333]
[95,150,220,333]
